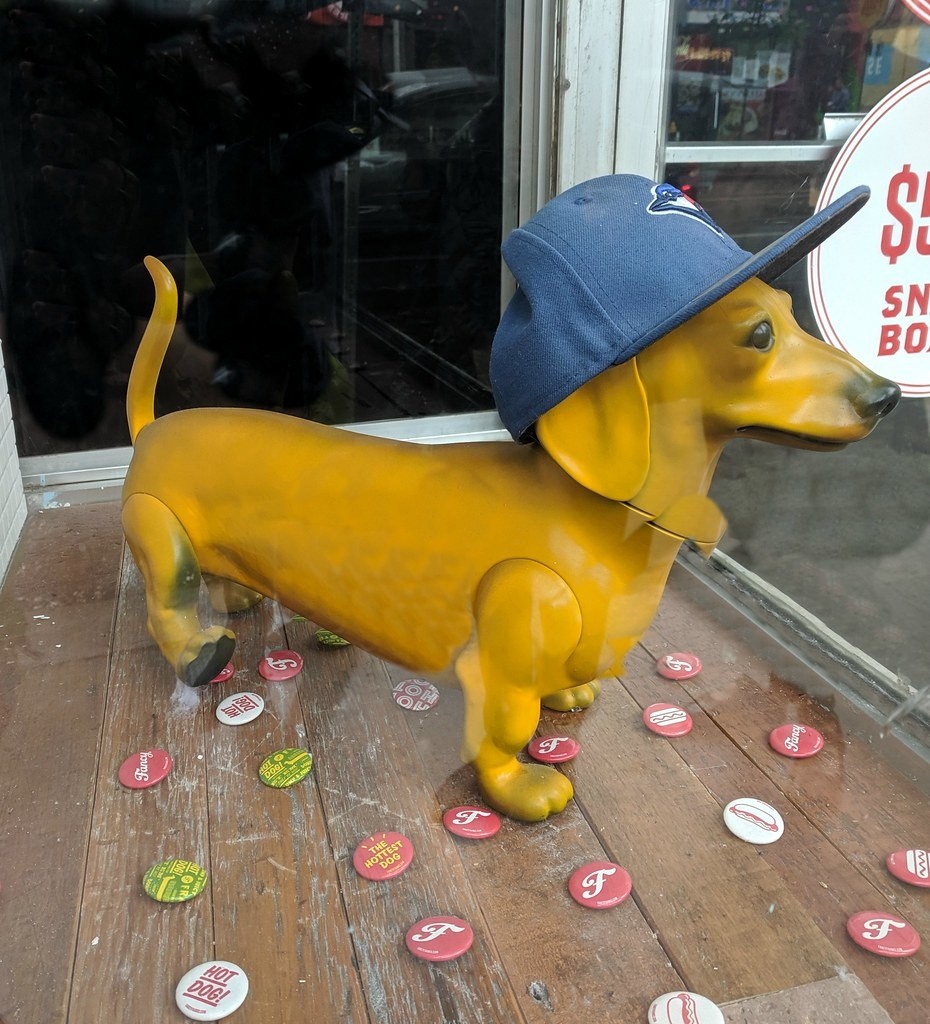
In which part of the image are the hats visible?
[489,174,871,449]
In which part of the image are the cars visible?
[313,73,503,291]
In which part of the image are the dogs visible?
[122,172,904,823]
